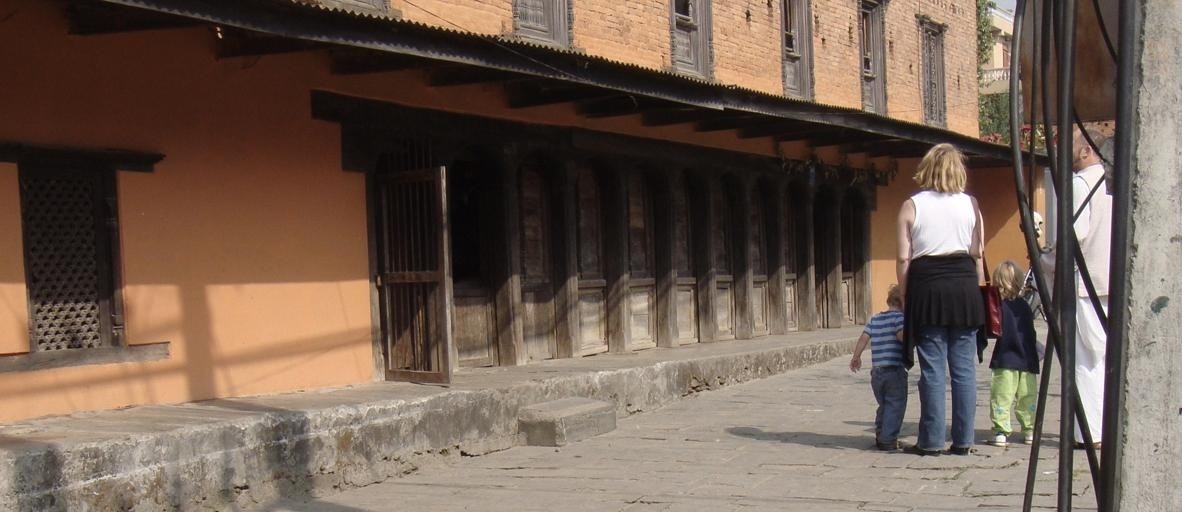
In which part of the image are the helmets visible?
[1019,212,1043,238]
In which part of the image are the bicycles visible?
[1017,266,1050,326]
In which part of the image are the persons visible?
[1035,127,1121,451]
[895,143,985,454]
[978,260,1040,447]
[849,286,907,451]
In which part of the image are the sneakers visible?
[875,433,903,450]
[1025,436,1033,444]
[987,435,1006,447]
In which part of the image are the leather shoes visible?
[904,446,939,456]
[951,444,969,455]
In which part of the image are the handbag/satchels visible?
[980,286,1003,339]
